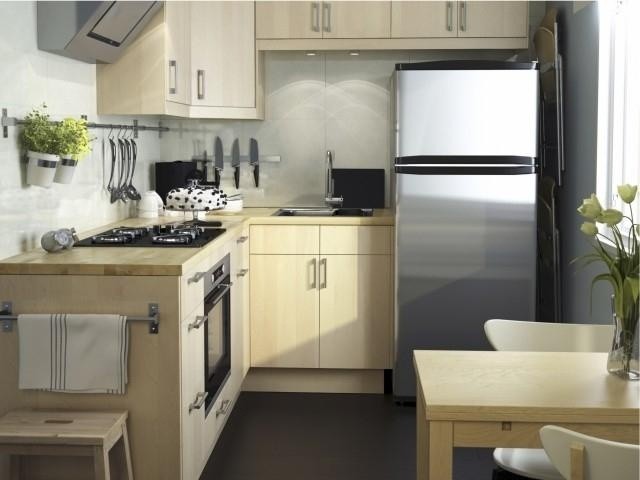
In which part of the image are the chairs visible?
[484,317,617,480]
[540,424,640,480]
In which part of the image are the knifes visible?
[249,137,260,187]
[231,138,241,190]
[214,136,224,189]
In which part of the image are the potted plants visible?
[54,118,97,184]
[24,110,66,188]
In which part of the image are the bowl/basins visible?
[226,193,243,212]
[136,190,164,219]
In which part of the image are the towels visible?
[17,313,130,395]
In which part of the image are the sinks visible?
[270,208,373,217]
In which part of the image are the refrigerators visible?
[391,59,541,408]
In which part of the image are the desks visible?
[413,351,640,479]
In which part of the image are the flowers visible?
[572,185,640,372]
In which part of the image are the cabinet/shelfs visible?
[96,2,266,119]
[0,260,207,480]
[256,1,528,50]
[229,230,248,399]
[250,224,393,368]
[0,217,250,480]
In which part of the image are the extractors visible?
[36,1,163,66]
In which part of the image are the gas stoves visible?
[73,223,226,248]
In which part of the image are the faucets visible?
[324,150,344,202]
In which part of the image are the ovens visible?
[204,252,232,419]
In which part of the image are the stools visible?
[0,409,134,480]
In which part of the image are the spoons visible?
[120,138,131,203]
[127,138,143,200]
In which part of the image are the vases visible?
[607,295,640,380]
